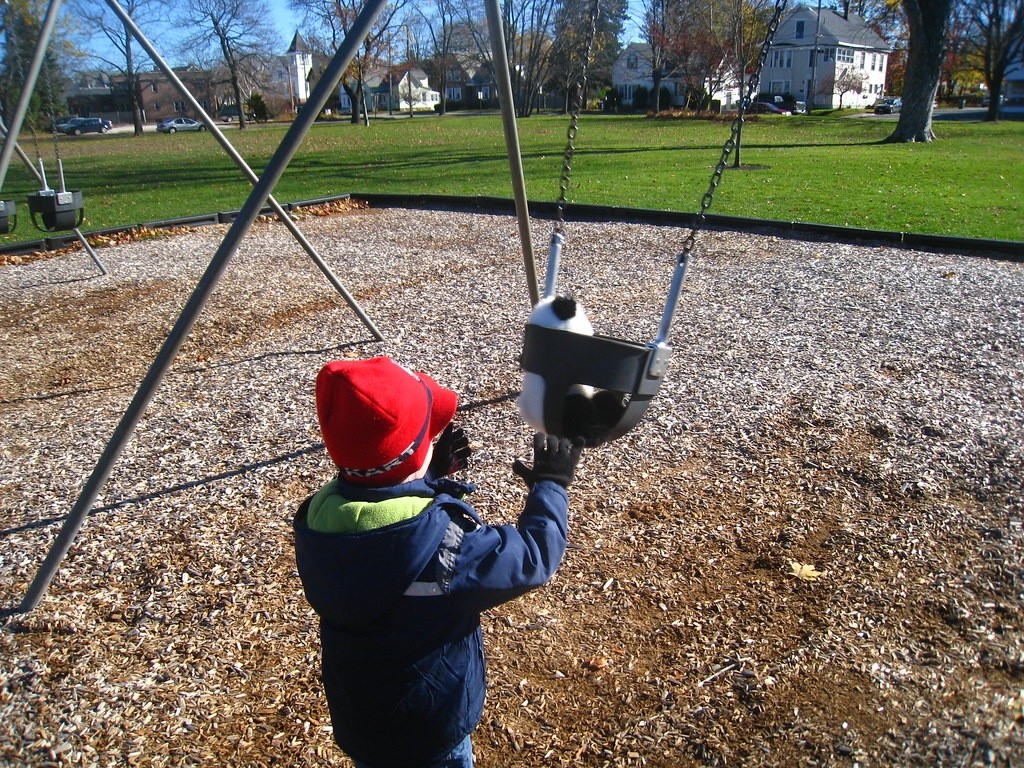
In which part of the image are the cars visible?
[981,95,992,107]
[43,115,113,137]
[218,115,255,122]
[156,117,207,134]
[742,102,791,116]
[874,98,903,114]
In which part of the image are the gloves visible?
[513,433,585,490]
[423,422,471,483]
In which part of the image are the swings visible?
[519,1,791,449]
[0,2,86,235]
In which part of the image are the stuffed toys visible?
[517,296,632,448]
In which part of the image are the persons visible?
[294,358,586,768]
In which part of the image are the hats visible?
[316,359,458,488]
